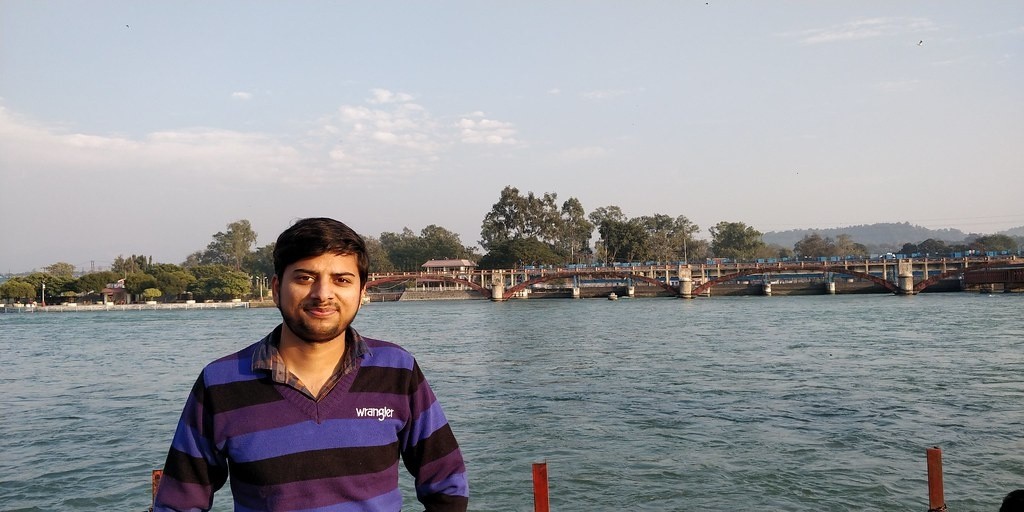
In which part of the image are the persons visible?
[999,489,1024,512]
[153,217,470,512]
